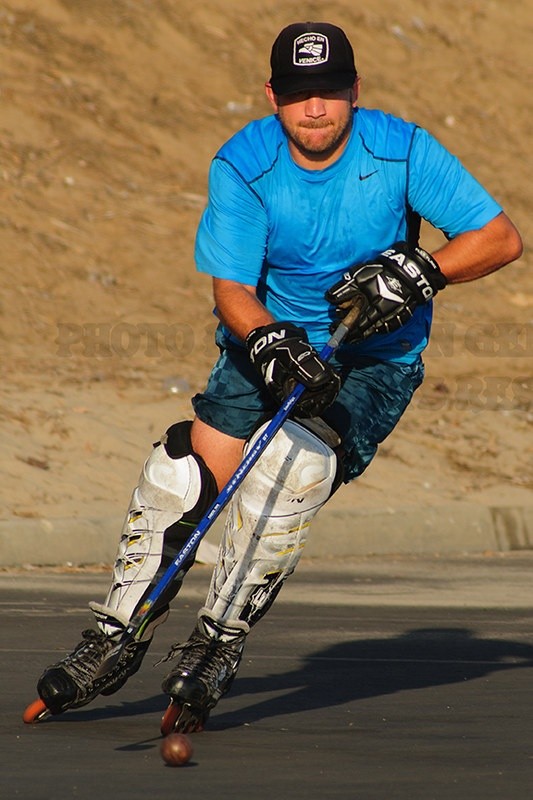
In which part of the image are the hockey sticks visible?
[93,300,361,680]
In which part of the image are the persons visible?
[23,22,523,734]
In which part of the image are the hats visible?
[268,21,357,96]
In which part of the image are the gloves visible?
[326,241,449,345]
[245,321,342,418]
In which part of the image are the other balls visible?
[160,732,193,765]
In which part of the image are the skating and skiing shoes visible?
[22,600,156,724]
[154,608,251,736]
